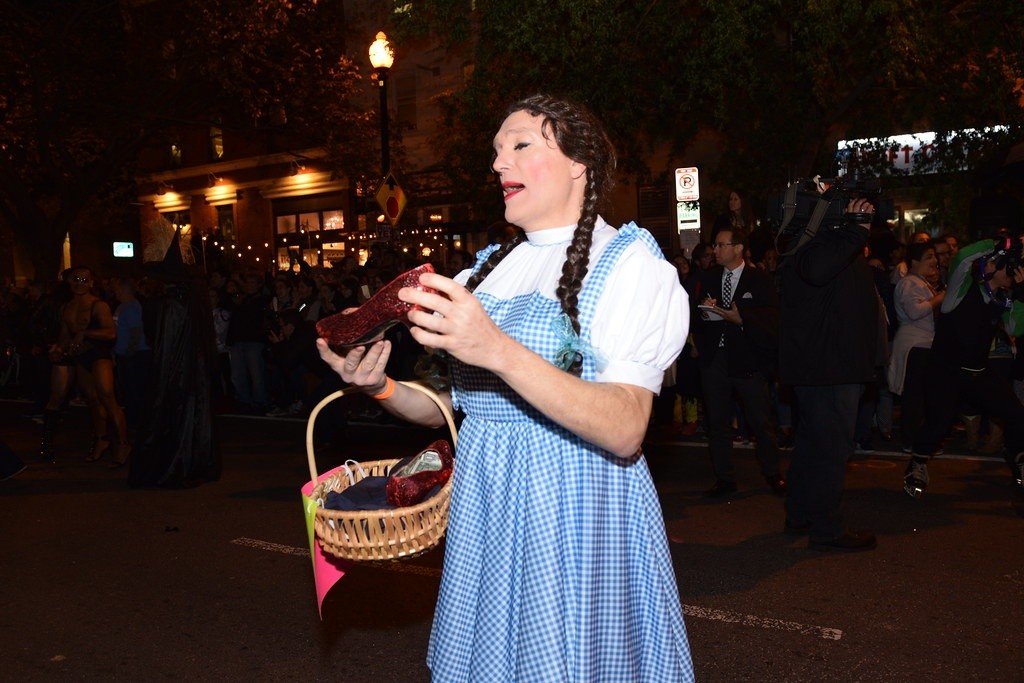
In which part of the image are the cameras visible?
[264,324,281,339]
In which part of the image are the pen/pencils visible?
[707,292,716,306]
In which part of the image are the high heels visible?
[85,435,113,463]
[107,440,133,468]
[314,263,436,347]
[387,440,454,507]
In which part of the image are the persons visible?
[316,94,693,683]
[648,169,1024,553]
[0,220,517,490]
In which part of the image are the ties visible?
[719,272,733,348]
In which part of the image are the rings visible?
[72,344,74,345]
[861,209,865,212]
[74,344,76,345]
[77,345,79,347]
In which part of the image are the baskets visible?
[306,381,459,561]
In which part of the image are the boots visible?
[38,408,57,464]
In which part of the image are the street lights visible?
[368,29,396,279]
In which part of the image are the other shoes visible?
[809,509,877,552]
[22,411,39,418]
[702,479,736,497]
[902,443,945,456]
[304,441,332,454]
[0,458,29,481]
[784,514,827,535]
[32,416,46,424]
[882,432,893,441]
[771,482,788,496]
[265,407,287,416]
[903,453,930,499]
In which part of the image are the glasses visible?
[712,243,735,249]
[71,276,90,283]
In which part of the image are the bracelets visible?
[366,377,394,399]
